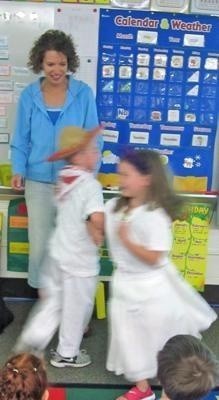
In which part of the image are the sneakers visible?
[114,383,156,400]
[49,347,92,369]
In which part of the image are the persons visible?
[10,31,98,336]
[1,356,50,400]
[156,334,218,398]
[15,126,104,370]
[87,148,218,399]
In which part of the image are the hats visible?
[47,122,105,161]
